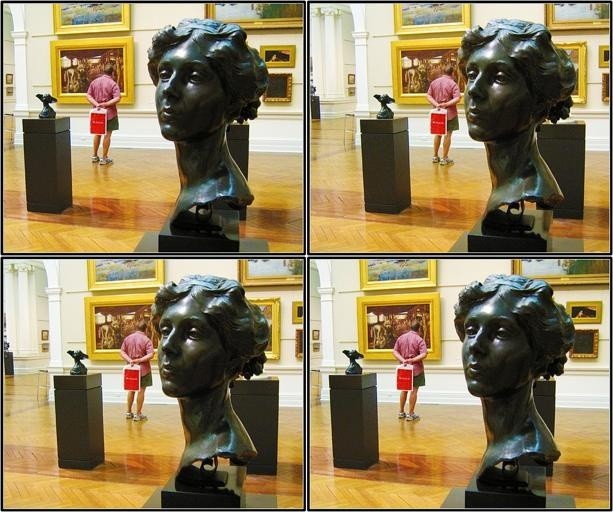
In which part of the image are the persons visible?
[101,319,158,349]
[87,66,121,165]
[454,276,574,489]
[66,63,116,93]
[407,64,457,93]
[393,320,428,421]
[458,20,575,235]
[575,310,583,318]
[148,19,268,236]
[120,322,154,421]
[270,53,278,61]
[372,318,423,346]
[427,66,460,167]
[149,276,269,491]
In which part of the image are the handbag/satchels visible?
[89,108,108,135]
[429,108,448,136]
[122,363,141,392]
[395,364,414,391]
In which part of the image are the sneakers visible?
[91,156,112,165]
[398,412,418,421]
[432,156,454,166]
[125,411,145,422]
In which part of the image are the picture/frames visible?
[356,291,442,361]
[392,3,471,36]
[86,259,165,292]
[358,259,437,292]
[259,44,296,68]
[204,3,304,30]
[262,72,292,102]
[510,259,610,358]
[390,38,466,105]
[84,293,160,361]
[543,3,610,31]
[52,3,131,36]
[50,36,135,105]
[237,259,304,360]
[552,40,610,103]
